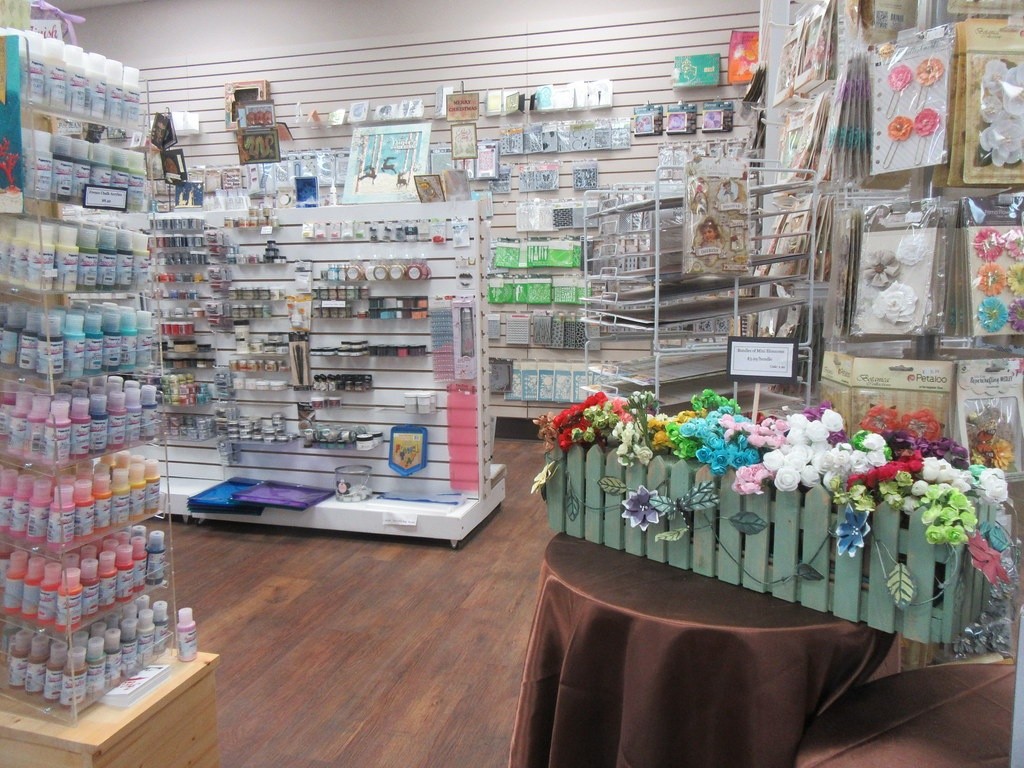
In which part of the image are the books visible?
[727,28,760,84]
[150,112,187,185]
[673,53,720,89]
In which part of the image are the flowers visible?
[528,389,1015,610]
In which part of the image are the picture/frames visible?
[235,100,276,130]
[444,170,471,201]
[342,122,431,206]
[237,129,281,165]
[414,176,445,204]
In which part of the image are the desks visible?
[793,664,1024,768]
[511,534,900,768]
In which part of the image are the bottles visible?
[176,607,196,662]
[1,25,168,708]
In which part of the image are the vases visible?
[548,440,1009,644]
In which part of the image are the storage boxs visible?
[221,79,269,131]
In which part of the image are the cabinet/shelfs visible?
[582,158,818,416]
[0,28,508,718]
